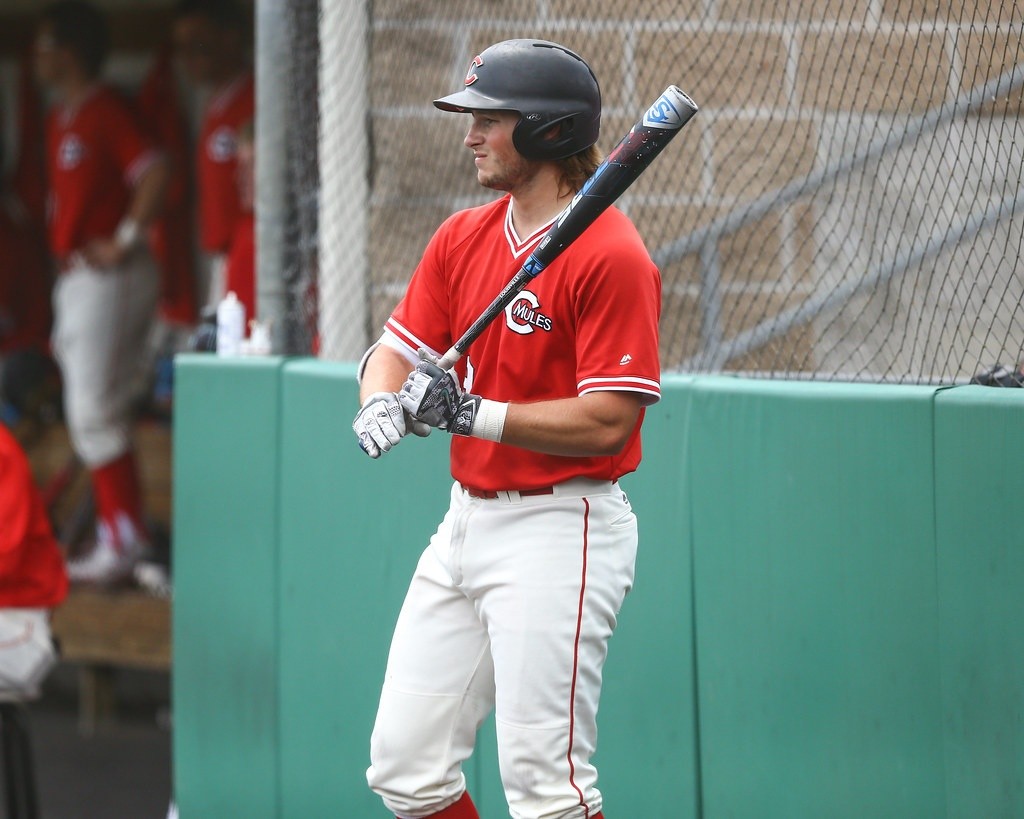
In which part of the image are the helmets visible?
[431,39,601,159]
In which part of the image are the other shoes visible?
[66,535,154,585]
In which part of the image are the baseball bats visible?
[358,84,699,456]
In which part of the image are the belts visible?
[461,483,551,499]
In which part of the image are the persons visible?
[0,426,67,707]
[349,38,664,818]
[176,3,257,321]
[33,15,171,587]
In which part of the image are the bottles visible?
[215,292,245,358]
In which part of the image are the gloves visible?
[398,348,482,437]
[351,391,432,459]
